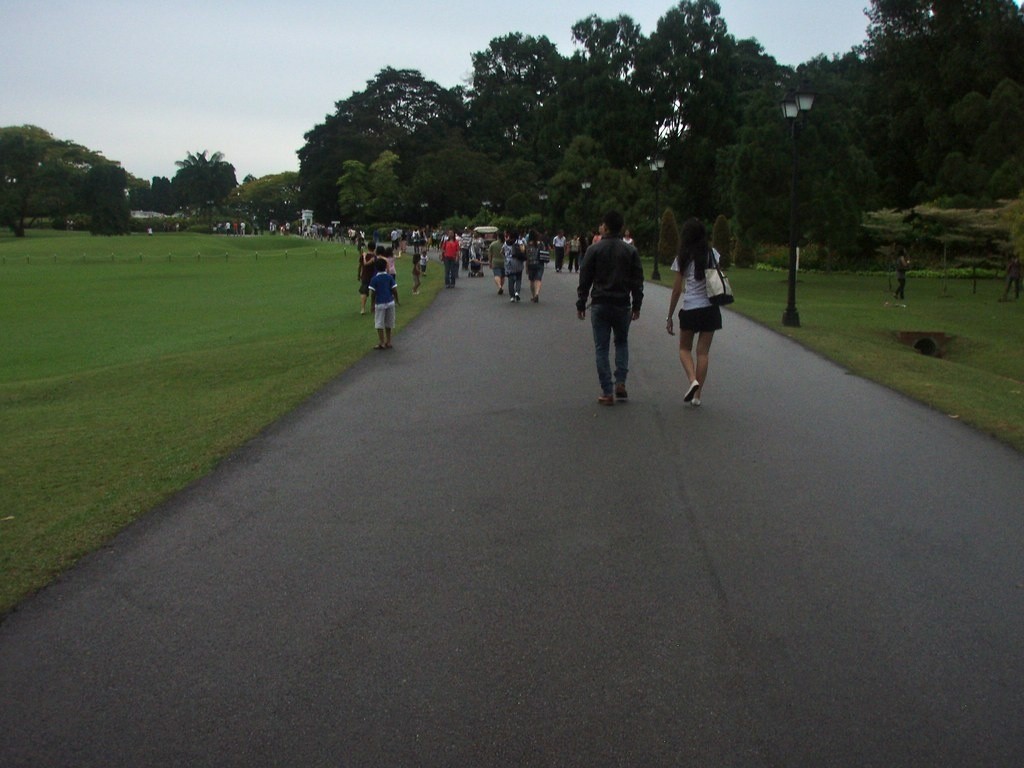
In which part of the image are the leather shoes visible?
[598,396,614,405]
[615,384,628,398]
[691,398,702,405]
[684,380,699,402]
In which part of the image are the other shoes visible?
[498,288,503,294]
[510,292,520,301]
[413,291,420,295]
[214,231,356,245]
[445,284,455,288]
[394,244,491,271]
[569,270,578,273]
[531,295,538,303]
[367,306,375,310]
[556,267,560,272]
[360,311,366,316]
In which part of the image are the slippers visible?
[374,344,392,349]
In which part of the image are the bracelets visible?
[666,317,673,321]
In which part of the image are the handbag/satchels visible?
[538,250,550,262]
[704,268,734,305]
[512,243,527,261]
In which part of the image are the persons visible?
[576,212,644,404]
[162,221,183,233]
[459,226,472,270]
[579,231,591,257]
[147,225,153,236]
[525,229,545,302]
[411,254,422,295]
[233,220,239,237]
[467,230,485,258]
[893,247,910,300]
[362,246,390,312]
[552,230,568,272]
[254,219,444,255]
[420,252,428,276]
[568,234,579,273]
[1002,256,1021,299]
[592,225,605,243]
[239,221,246,237]
[518,229,551,253]
[441,230,460,288]
[665,221,720,406]
[67,218,73,232]
[225,220,231,237]
[385,247,402,281]
[500,230,527,302]
[356,242,376,314]
[621,230,634,246]
[488,231,507,295]
[368,258,397,348]
